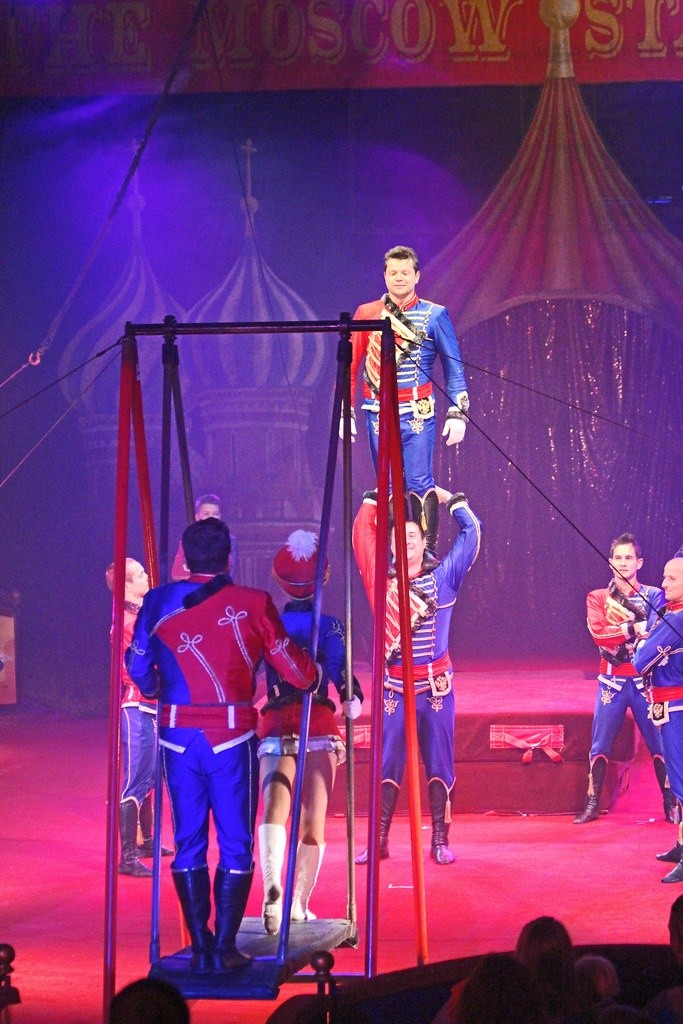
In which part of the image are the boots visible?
[171,861,216,975]
[652,753,683,823]
[355,779,400,867]
[118,790,174,881]
[257,823,286,935]
[387,487,441,578]
[208,862,253,975]
[573,751,610,824]
[289,841,328,920]
[424,777,458,865]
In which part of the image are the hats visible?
[270,530,330,602]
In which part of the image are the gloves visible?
[314,662,322,694]
[342,695,363,720]
[337,407,358,444]
[441,406,470,448]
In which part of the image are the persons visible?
[108,977,191,1024]
[105,494,364,977]
[441,895,683,1024]
[333,245,470,579]
[351,484,482,866]
[573,533,683,883]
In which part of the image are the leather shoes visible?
[661,864,683,884]
[653,842,683,862]
